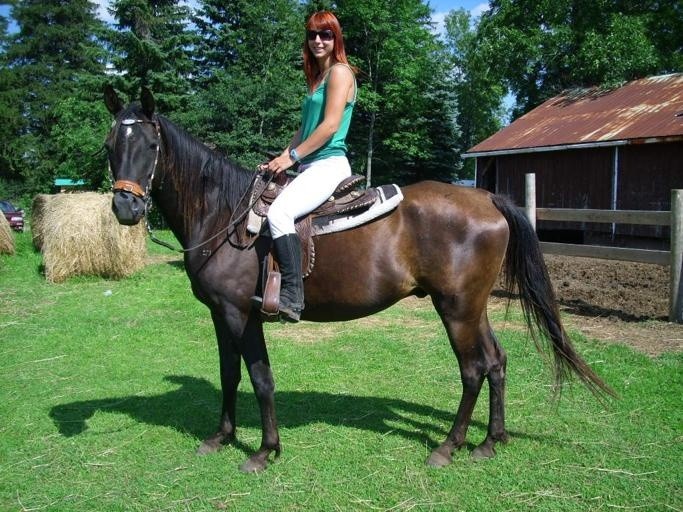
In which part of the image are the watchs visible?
[289,148,301,164]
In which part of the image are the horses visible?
[102,83,620,476]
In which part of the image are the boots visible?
[250,233,304,323]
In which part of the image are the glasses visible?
[306,30,333,40]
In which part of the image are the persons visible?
[251,12,362,324]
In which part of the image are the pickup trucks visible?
[0,201,24,232]
